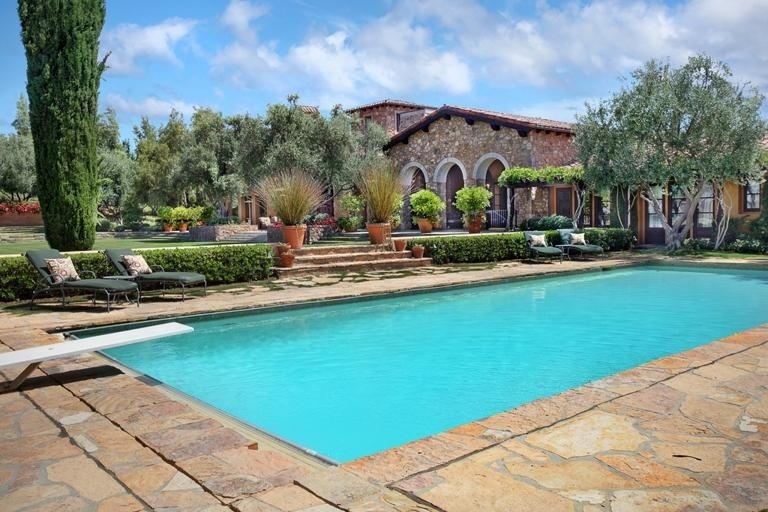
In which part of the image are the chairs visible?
[19,246,140,314]
[555,225,607,263]
[522,229,563,265]
[97,246,209,305]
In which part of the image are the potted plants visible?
[154,204,211,233]
[245,166,333,268]
[336,163,493,259]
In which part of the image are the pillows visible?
[528,234,549,249]
[119,253,153,275]
[569,232,587,246]
[42,256,83,282]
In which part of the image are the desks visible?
[102,275,140,305]
